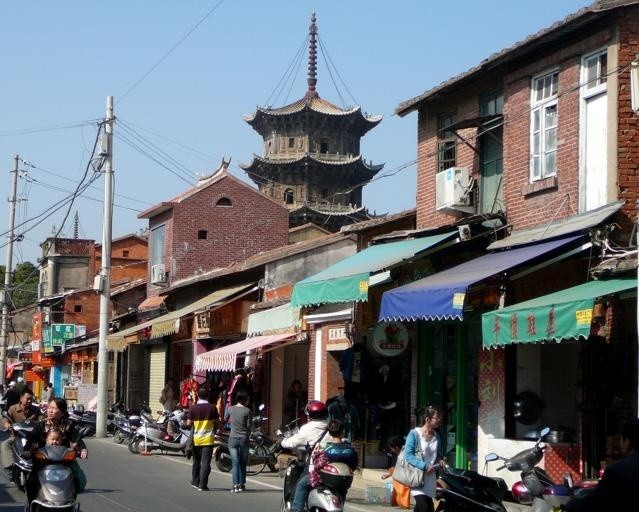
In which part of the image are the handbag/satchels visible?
[390,479,413,510]
[391,428,425,490]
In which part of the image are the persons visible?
[0,377,89,506]
[281,400,359,512]
[529,400,572,444]
[186,387,253,493]
[560,418,639,512]
[159,377,179,413]
[381,406,447,512]
[286,380,308,419]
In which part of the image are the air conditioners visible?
[434,167,472,211]
[149,264,167,284]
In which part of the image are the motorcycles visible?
[277,429,354,512]
[0,398,279,512]
[434,427,605,512]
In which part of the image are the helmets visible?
[302,400,332,418]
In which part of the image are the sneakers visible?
[229,483,246,493]
[188,480,210,492]
